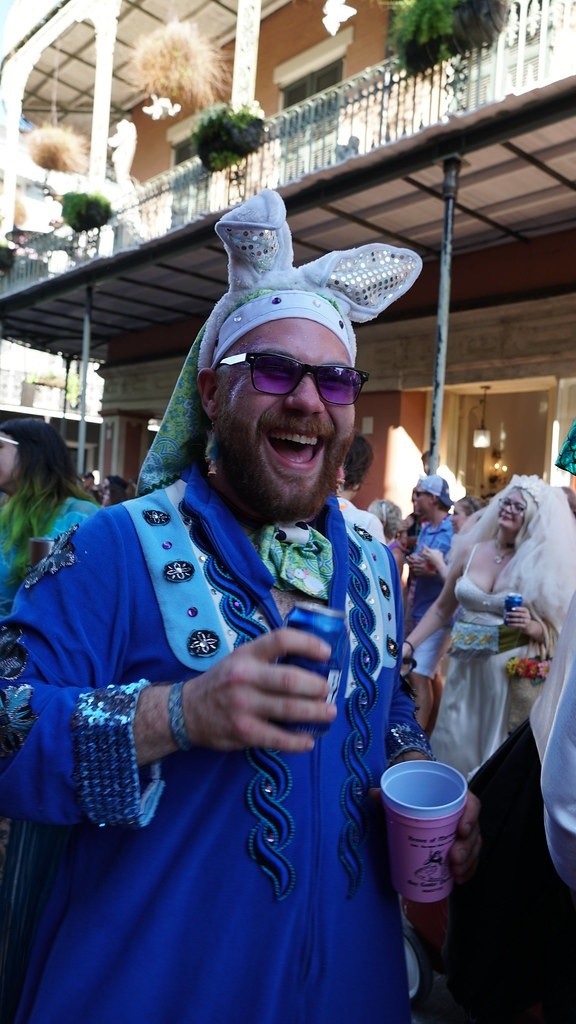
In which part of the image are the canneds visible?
[268,601,346,738]
[503,592,523,625]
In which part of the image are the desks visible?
[5,230,80,251]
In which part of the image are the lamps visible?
[472,383,492,449]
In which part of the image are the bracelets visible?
[167,681,194,753]
[404,640,414,657]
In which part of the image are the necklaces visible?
[494,537,513,564]
[418,514,450,548]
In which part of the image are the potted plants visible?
[189,99,269,179]
[22,119,89,174]
[0,241,16,272]
[381,0,510,74]
[59,191,113,232]
[127,17,226,107]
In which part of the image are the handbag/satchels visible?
[507,621,554,733]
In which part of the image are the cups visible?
[379,759,469,904]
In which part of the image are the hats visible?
[195,190,422,372]
[419,474,454,506]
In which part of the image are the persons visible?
[107,119,137,191]
[0,190,483,1024]
[0,419,139,614]
[447,590,576,1024]
[339,435,576,785]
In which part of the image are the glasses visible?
[416,490,432,498]
[498,498,527,515]
[216,352,370,406]
